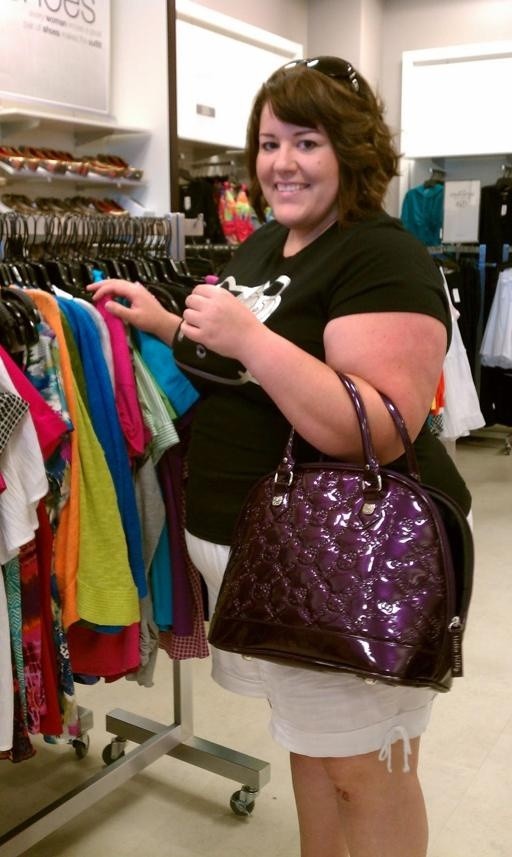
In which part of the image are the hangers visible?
[0,158,511,349]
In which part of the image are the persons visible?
[84,57,475,857]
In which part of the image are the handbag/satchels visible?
[206,374,474,695]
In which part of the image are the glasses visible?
[276,57,359,93]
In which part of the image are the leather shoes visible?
[0,146,143,179]
[0,194,129,217]
